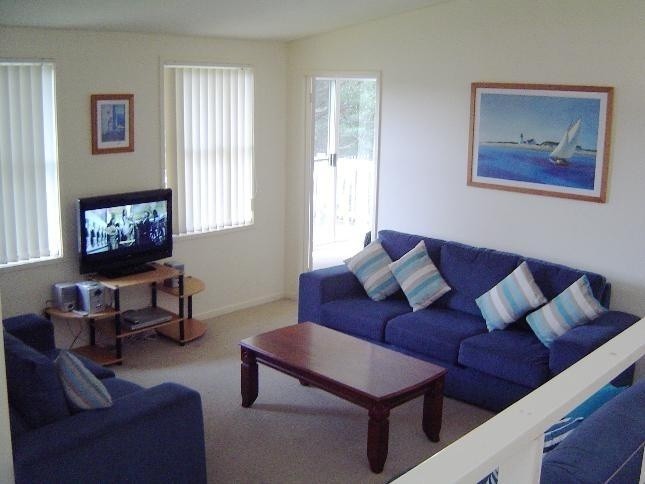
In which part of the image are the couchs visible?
[299,230,637,414]
[477,378,645,484]
[3,311,206,484]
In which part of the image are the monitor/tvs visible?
[78,188,173,278]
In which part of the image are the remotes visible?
[72,308,88,316]
[124,317,139,324]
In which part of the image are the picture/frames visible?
[91,94,136,154]
[465,83,614,205]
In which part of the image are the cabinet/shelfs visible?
[43,261,206,367]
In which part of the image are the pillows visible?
[55,350,115,415]
[479,416,584,484]
[562,382,629,421]
[344,238,609,351]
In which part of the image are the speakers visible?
[76,280,106,314]
[163,261,184,288]
[53,283,78,313]
